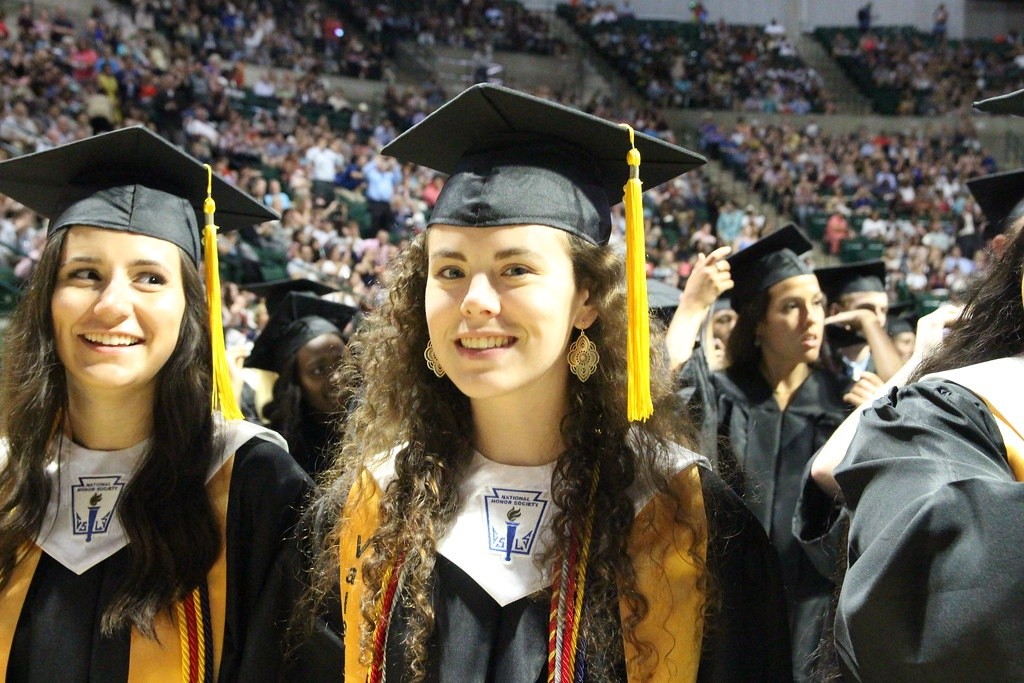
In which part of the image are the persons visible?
[0,126,342,683]
[306,82,802,683]
[0,0,1024,683]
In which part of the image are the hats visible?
[238,278,336,315]
[243,292,357,374]
[884,303,918,336]
[619,275,682,327]
[379,83,708,422]
[964,169,1024,236]
[724,224,816,301]
[811,261,889,297]
[0,125,280,418]
